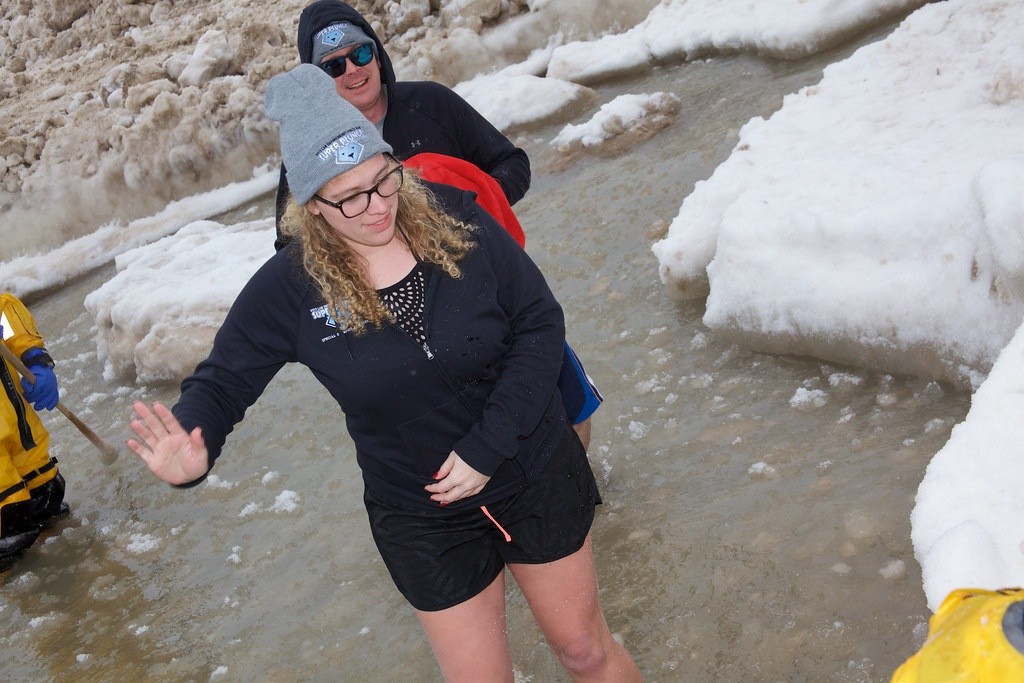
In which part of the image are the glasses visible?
[317,41,374,78]
[310,152,404,218]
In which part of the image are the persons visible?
[127,63,645,683]
[0,287,74,576]
[273,0,604,457]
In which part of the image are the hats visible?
[263,64,392,206]
[310,22,381,66]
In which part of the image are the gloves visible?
[20,347,59,411]
